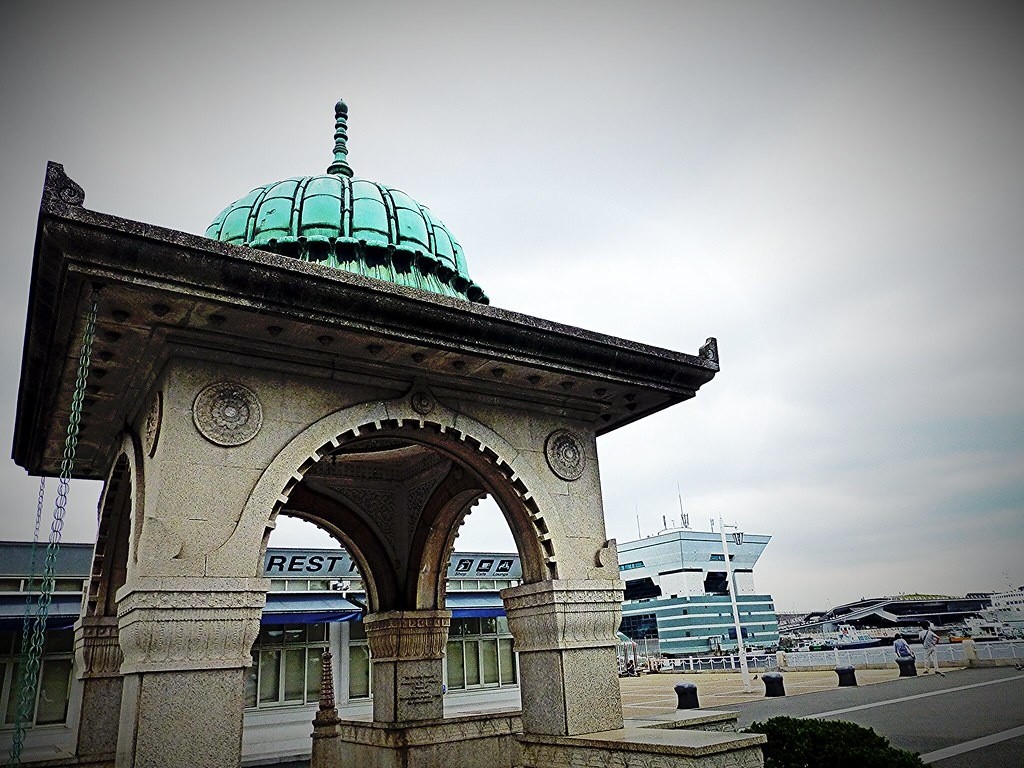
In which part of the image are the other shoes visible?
[935,670,942,674]
[923,669,929,674]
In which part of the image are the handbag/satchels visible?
[899,644,912,657]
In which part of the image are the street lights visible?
[718,512,754,694]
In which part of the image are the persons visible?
[891,632,920,678]
[627,659,635,674]
[917,621,943,674]
[840,627,848,634]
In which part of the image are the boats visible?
[946,589,1024,644]
[777,623,883,650]
